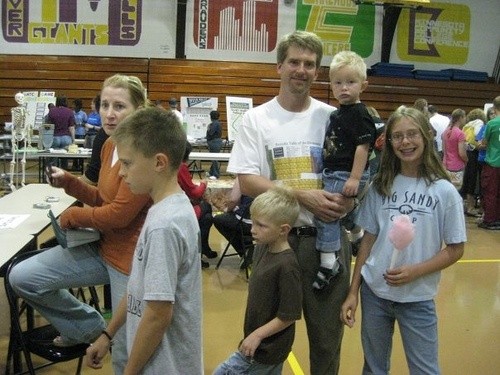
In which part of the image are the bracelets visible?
[102,330,114,353]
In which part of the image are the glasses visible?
[389,129,421,142]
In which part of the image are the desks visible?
[190,179,235,212]
[0,184,83,331]
[188,152,232,179]
[0,134,39,155]
[0,234,35,375]
[0,148,93,184]
[40,139,85,175]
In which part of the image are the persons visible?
[8,31,500,375]
[311,51,378,294]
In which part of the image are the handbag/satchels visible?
[450,175,463,185]
[462,125,481,149]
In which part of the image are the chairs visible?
[215,198,254,279]
[4,251,112,375]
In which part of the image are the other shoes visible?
[54,334,82,346]
[206,171,210,176]
[209,176,216,179]
[477,220,500,229]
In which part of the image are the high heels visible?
[201,260,210,268]
[201,246,218,258]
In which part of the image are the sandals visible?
[312,259,343,293]
[351,236,364,257]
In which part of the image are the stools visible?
[188,169,205,180]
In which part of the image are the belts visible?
[289,225,317,238]
